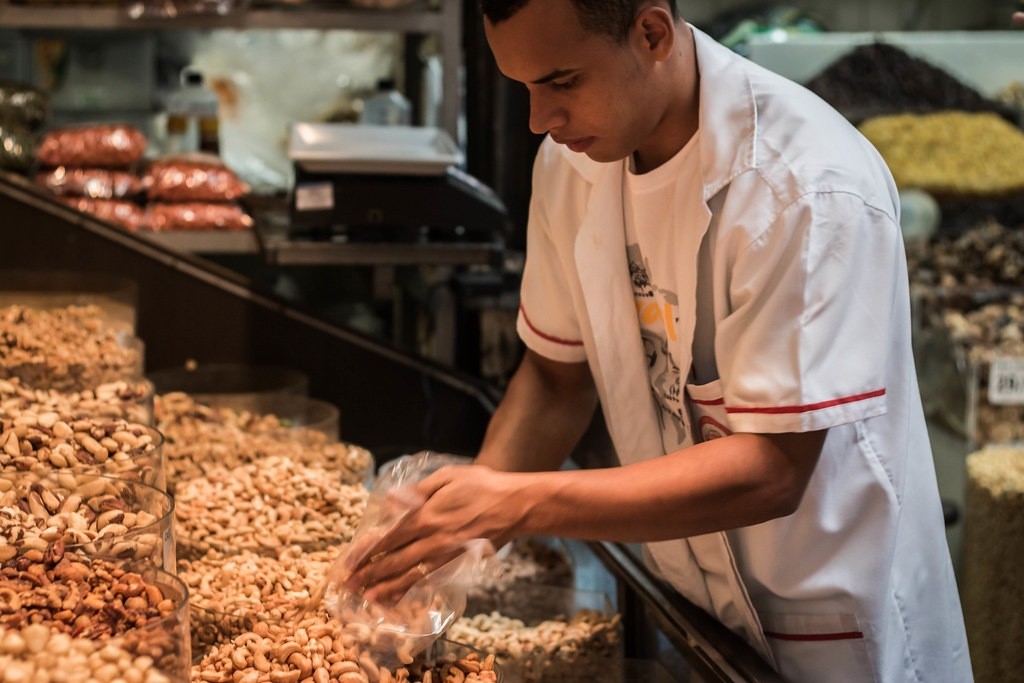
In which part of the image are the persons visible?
[333,0,978,683]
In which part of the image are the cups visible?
[201,387,338,441]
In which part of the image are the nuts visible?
[0,299,620,683]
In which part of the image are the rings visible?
[418,561,430,578]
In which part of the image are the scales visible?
[287,121,506,243]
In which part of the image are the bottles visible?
[354,77,409,126]
[157,66,222,153]
